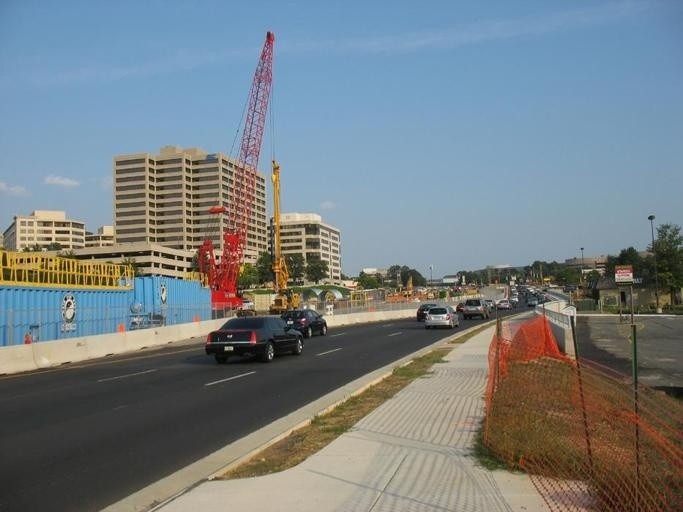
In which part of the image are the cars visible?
[462,298,490,320]
[206,316,305,364]
[423,306,460,329]
[279,309,327,338]
[409,285,546,313]
[416,303,442,322]
[238,299,254,309]
[562,285,574,293]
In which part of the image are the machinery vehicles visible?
[197,31,275,317]
[269,159,299,314]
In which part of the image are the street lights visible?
[647,215,663,314]
[429,267,432,289]
[580,247,584,269]
